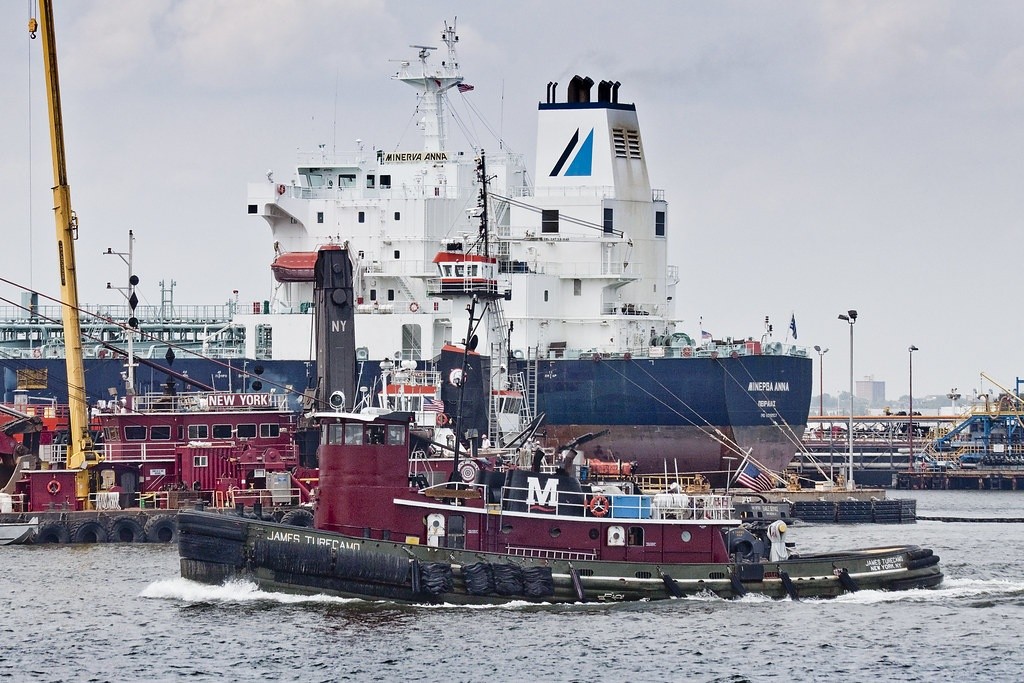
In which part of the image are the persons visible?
[524,438,545,465]
[482,435,491,451]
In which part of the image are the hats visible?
[482,434,487,438]
[527,437,532,441]
[536,441,540,444]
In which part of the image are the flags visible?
[702,331,713,339]
[790,314,797,339]
[424,398,444,413]
[737,462,774,493]
[457,83,474,93]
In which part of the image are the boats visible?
[0,233,612,544]
[176,147,943,607]
[0,0,812,484]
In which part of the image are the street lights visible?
[836,311,856,491]
[814,345,830,437]
[909,345,919,473]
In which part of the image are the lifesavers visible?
[36,520,68,546]
[590,496,610,516]
[69,519,108,547]
[920,462,927,470]
[145,512,180,545]
[282,509,315,526]
[410,301,419,312]
[683,347,691,356]
[108,517,145,545]
[278,184,286,195]
[435,413,447,426]
[47,480,62,494]
